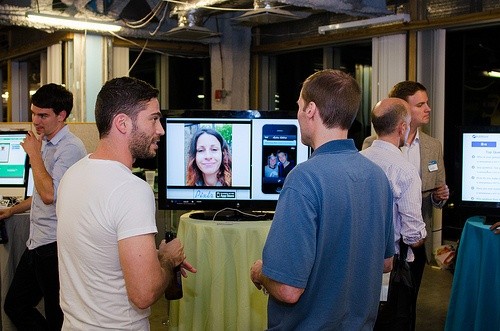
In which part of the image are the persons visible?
[186,128,232,186]
[56,77,197,331]
[358,98,427,331]
[278,150,296,183]
[265,153,280,177]
[0,83,88,331]
[250,68,396,331]
[361,80,449,331]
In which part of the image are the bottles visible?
[164,231,183,300]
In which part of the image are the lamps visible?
[25,0,125,32]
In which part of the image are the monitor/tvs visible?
[456,125,500,226]
[0,130,32,202]
[159,109,315,222]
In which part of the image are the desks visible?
[443,215,500,331]
[0,203,46,331]
[168,209,275,331]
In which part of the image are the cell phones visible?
[261,124,297,194]
